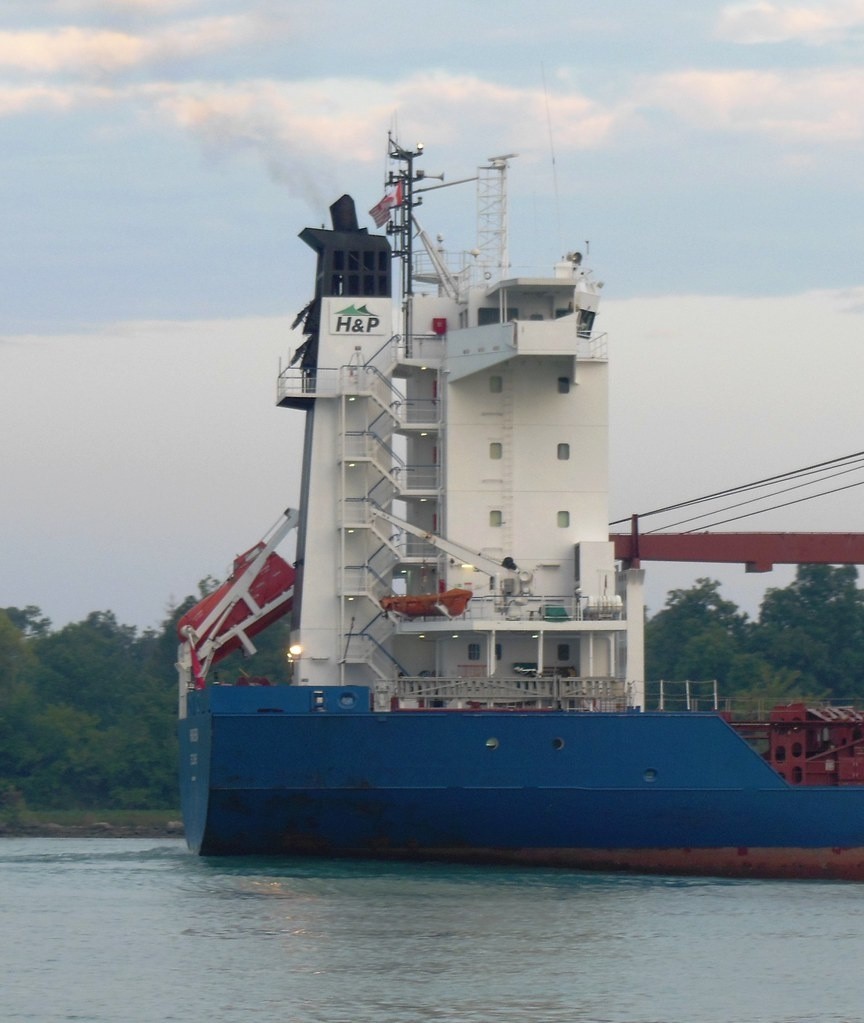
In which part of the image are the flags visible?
[369,182,401,229]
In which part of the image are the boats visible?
[177,128,864,881]
[380,588,473,616]
